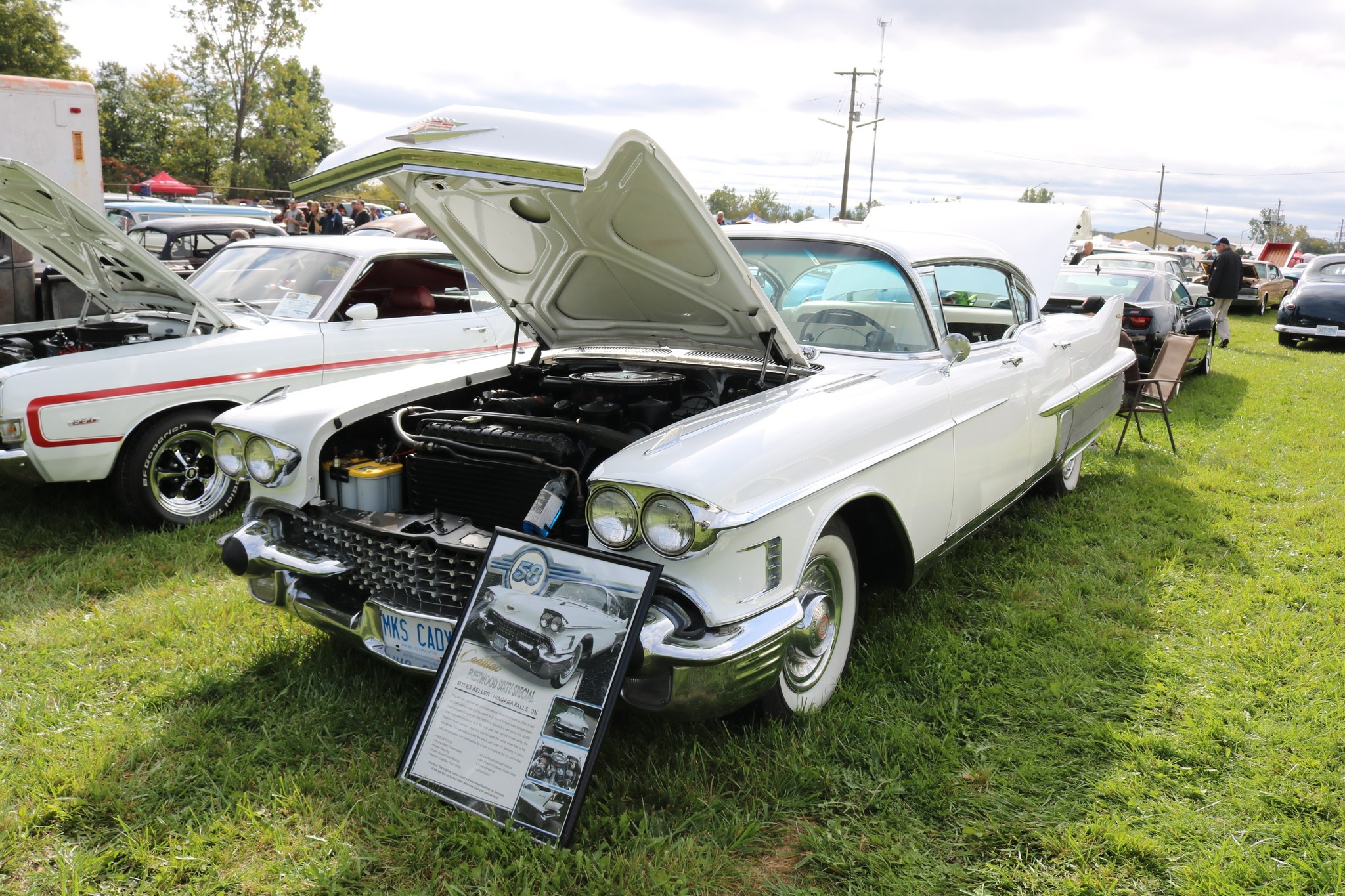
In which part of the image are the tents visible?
[1091,234,1219,255]
[713,214,726,219]
[129,171,197,195]
[739,212,771,223]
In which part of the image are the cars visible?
[35,217,294,323]
[1067,252,1214,316]
[1054,264,1217,393]
[104,189,441,241]
[1071,236,1319,283]
[1273,254,1345,346]
[0,156,529,526]
[1197,258,1296,316]
[207,107,1140,725]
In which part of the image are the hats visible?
[1211,237,1230,245]
[394,203,406,209]
[376,209,381,212]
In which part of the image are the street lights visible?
[1322,238,1341,254]
[1131,201,1161,250]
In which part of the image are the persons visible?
[991,296,1012,310]
[1176,245,1183,252]
[207,194,415,261]
[929,290,961,305]
[1182,248,1186,252]
[1168,246,1175,252]
[715,211,725,225]
[1069,241,1095,265]
[833,216,839,221]
[1206,237,1243,348]
[1071,295,1143,413]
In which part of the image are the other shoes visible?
[1219,338,1229,348]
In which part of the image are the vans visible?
[106,202,274,239]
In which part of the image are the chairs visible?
[311,280,352,321]
[1114,331,1199,460]
[382,285,442,316]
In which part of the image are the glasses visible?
[1215,245,1220,246]
[325,205,331,208]
[371,208,374,210]
[289,202,294,204]
